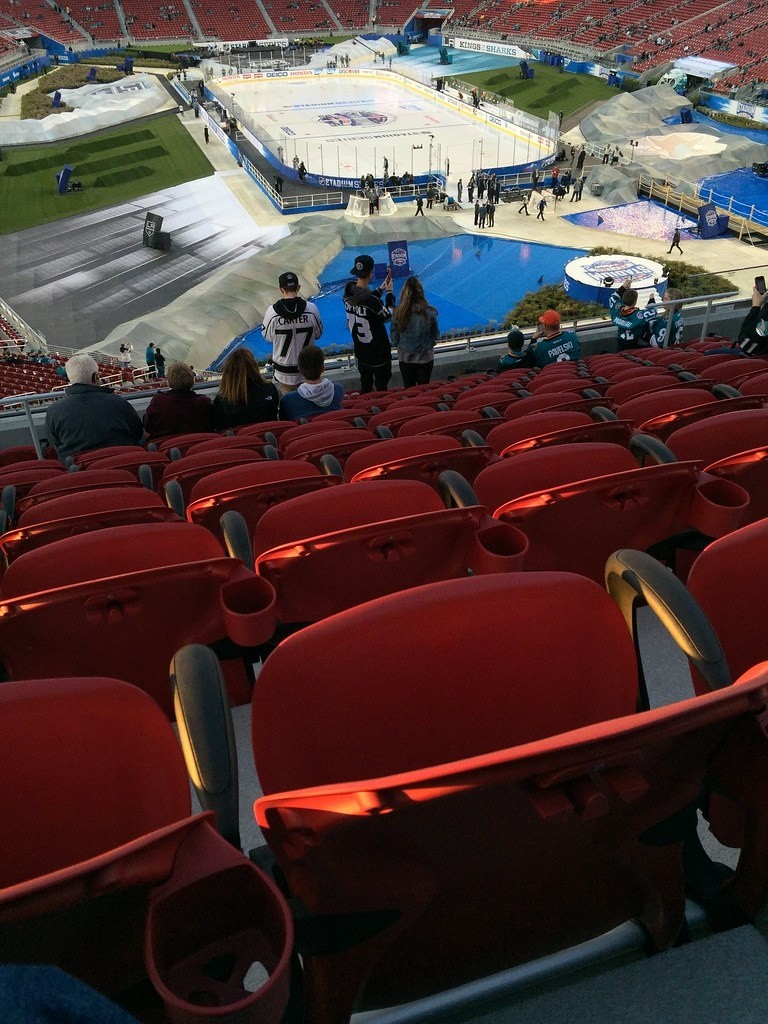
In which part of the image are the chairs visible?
[0,313,768,1024]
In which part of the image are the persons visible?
[213,348,279,432]
[704,286,768,357]
[189,366,198,377]
[698,221,702,235]
[0,0,768,229]
[143,361,216,442]
[146,343,156,380]
[261,271,323,395]
[341,256,395,395]
[495,331,533,373]
[120,342,133,366]
[667,228,683,255]
[0,342,70,382]
[280,345,345,421]
[45,355,143,466]
[608,280,684,353]
[154,348,165,380]
[390,277,440,390]
[527,309,580,369]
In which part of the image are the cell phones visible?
[754,276,767,295]
[387,268,392,283]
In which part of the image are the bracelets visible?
[386,290,393,293]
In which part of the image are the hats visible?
[539,310,560,325]
[350,255,374,277]
[278,272,298,286]
